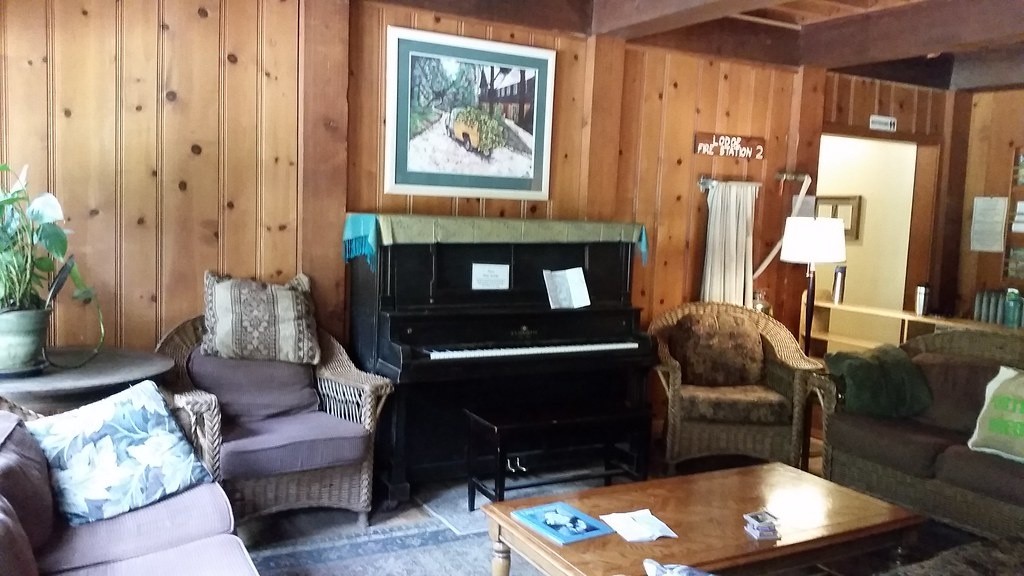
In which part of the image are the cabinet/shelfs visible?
[799,288,1024,352]
[1000,144,1024,285]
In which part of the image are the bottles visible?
[1005,289,1019,327]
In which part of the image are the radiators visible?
[973,289,1005,325]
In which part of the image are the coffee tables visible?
[478,461,923,576]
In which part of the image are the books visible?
[510,500,616,546]
[542,267,591,310]
[598,508,679,543]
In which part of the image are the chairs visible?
[648,300,824,475]
[152,315,393,528]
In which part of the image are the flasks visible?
[831,267,846,304]
[915,284,930,315]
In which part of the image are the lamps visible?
[779,217,847,356]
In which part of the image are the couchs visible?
[812,328,1024,550]
[0,390,256,576]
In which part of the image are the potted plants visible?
[0,162,106,376]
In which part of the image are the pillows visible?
[669,313,767,387]
[24,381,214,527]
[967,365,1024,464]
[824,343,934,418]
[200,269,322,366]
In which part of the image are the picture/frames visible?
[384,25,557,201]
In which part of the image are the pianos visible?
[349,213,658,513]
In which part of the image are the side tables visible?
[0,346,176,417]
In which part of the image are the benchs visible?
[460,394,653,511]
